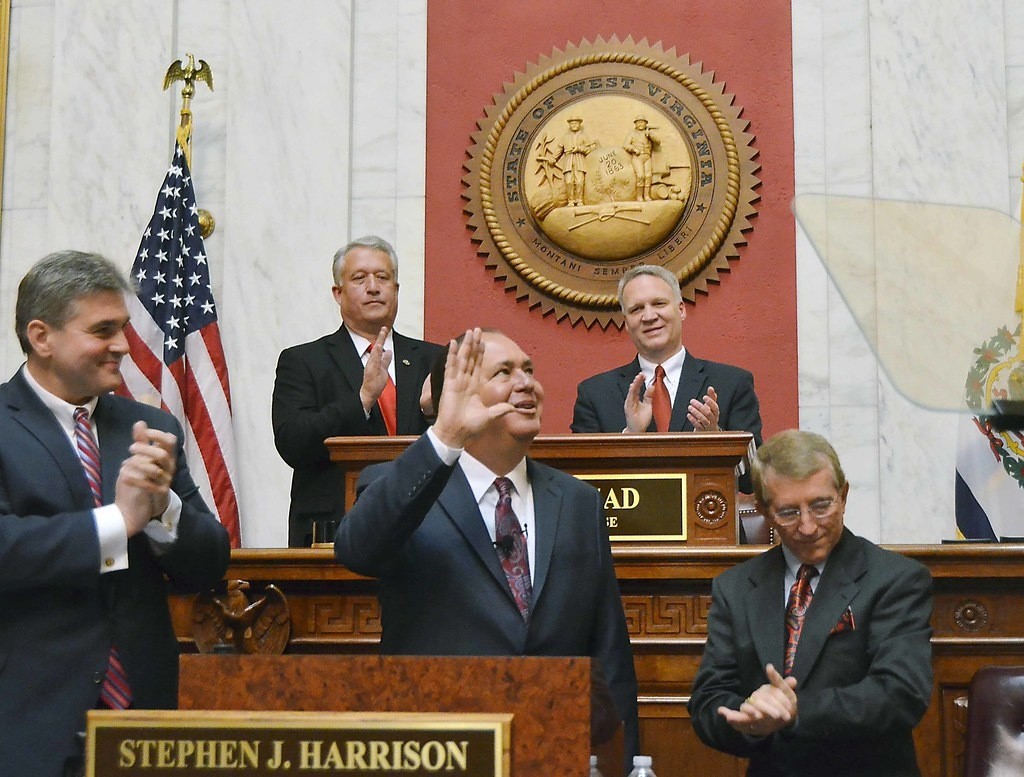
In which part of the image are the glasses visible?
[765,483,846,528]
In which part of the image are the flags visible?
[112,145,243,548]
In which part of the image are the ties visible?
[495,476,535,622]
[653,363,671,432]
[74,406,134,713]
[784,563,821,679]
[367,342,397,437]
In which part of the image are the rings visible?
[156,469,164,478]
[705,421,710,428]
[745,697,752,703]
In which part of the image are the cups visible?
[311,520,340,543]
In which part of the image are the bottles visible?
[628,756,657,777]
[590,756,603,777]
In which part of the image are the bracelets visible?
[421,409,436,417]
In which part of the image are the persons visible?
[547,117,591,205]
[687,427,936,777]
[0,251,230,777]
[569,264,761,545]
[272,235,449,548]
[623,114,661,201]
[334,327,639,777]
